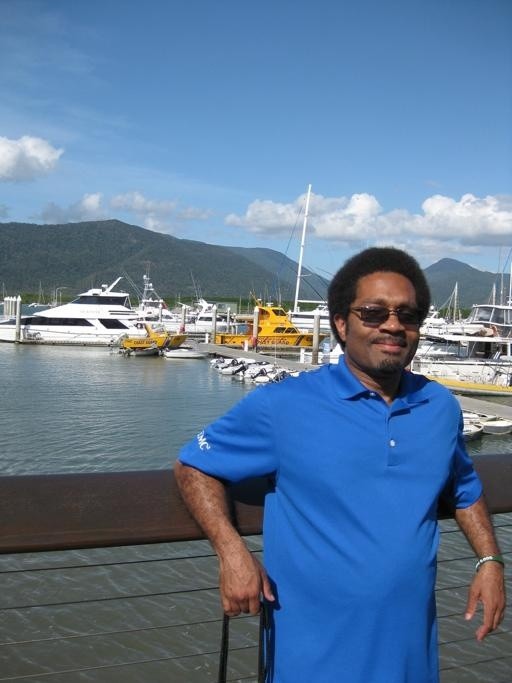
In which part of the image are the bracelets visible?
[476,553,507,576]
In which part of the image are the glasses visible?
[350,307,423,324]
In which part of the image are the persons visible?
[174,245,507,682]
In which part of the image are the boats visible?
[404,246,512,397]
[461,408,512,442]
